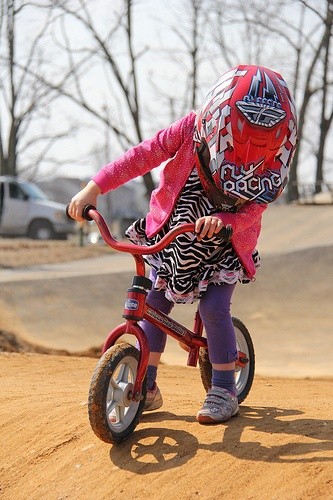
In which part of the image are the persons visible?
[67,64,296,426]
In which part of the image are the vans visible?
[0,175,83,242]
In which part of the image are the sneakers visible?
[196,385,240,424]
[108,382,163,422]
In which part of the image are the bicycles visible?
[66,202,255,445]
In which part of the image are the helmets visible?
[192,65,299,201]
